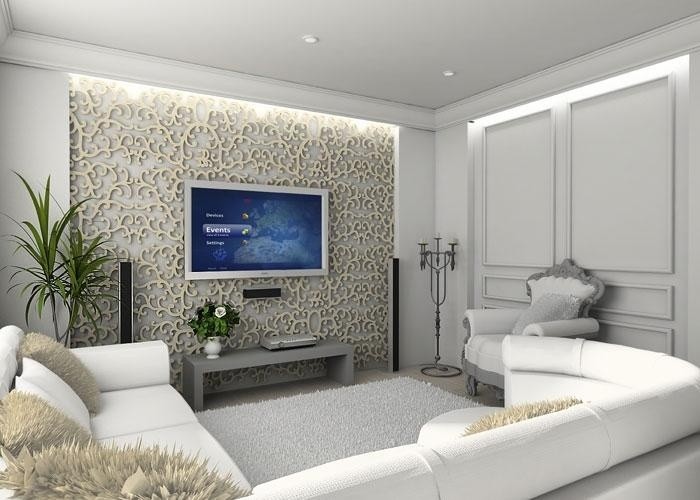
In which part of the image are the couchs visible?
[0,326,699,500]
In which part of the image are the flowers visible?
[187,301,241,343]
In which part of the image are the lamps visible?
[417,231,463,378]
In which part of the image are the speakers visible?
[243,288,283,300]
[118,261,134,343]
[387,257,399,370]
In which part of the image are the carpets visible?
[193,375,489,488]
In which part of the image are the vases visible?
[203,337,221,360]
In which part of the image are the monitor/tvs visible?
[183,180,328,280]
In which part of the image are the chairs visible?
[458,259,607,399]
